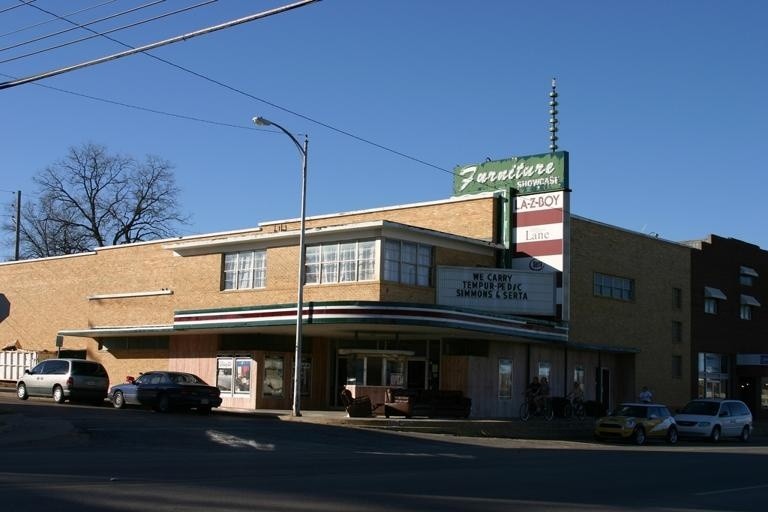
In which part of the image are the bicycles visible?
[519,391,586,425]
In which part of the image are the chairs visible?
[341,388,372,417]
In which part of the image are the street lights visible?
[251,116,308,417]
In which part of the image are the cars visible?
[595,399,753,446]
[16,358,222,413]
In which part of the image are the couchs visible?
[384,387,472,419]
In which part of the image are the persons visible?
[523,376,553,414]
[638,386,655,403]
[566,381,584,417]
